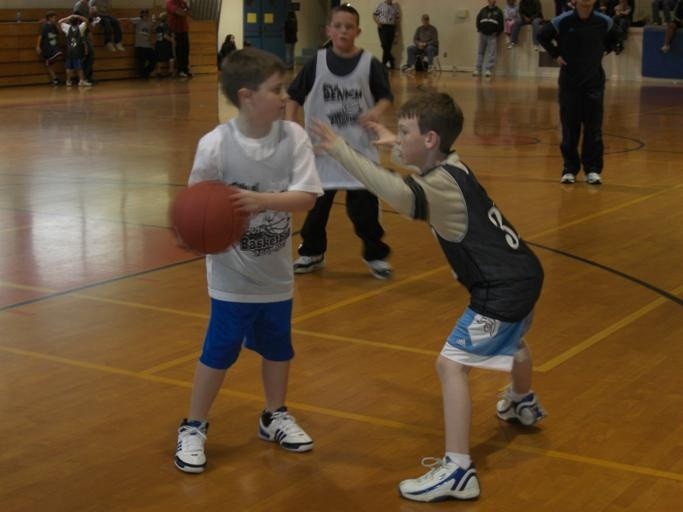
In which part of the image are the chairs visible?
[423,40,442,73]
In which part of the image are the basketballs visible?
[170,180,248,251]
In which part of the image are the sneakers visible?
[294,254,325,273]
[116,42,126,51]
[362,256,391,280]
[106,42,116,52]
[583,172,601,185]
[399,456,480,504]
[495,383,548,428]
[258,406,313,452]
[78,80,92,86]
[66,80,72,87]
[428,65,433,72]
[661,46,670,53]
[560,174,575,183]
[473,71,481,75]
[174,418,209,474]
[486,70,492,76]
[405,65,416,71]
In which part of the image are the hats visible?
[140,9,149,15]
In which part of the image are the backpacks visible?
[66,26,82,58]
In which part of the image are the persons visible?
[500,0,682,57]
[306,88,544,504]
[471,1,503,77]
[404,14,440,75]
[171,47,326,476]
[217,34,236,71]
[533,1,621,185]
[282,1,298,70]
[372,0,400,69]
[34,0,193,89]
[282,5,395,282]
